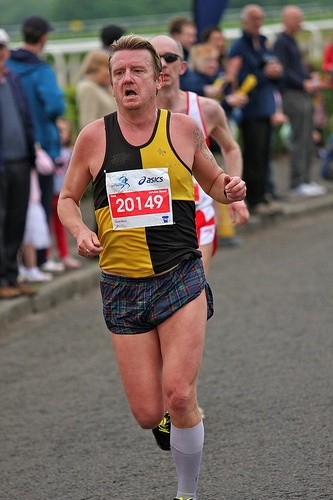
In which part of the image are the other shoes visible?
[173,497,193,500]
[60,256,80,268]
[41,261,65,272]
[19,265,52,281]
[16,283,38,296]
[289,182,326,197]
[0,287,21,298]
[253,201,288,216]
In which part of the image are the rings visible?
[85,248,89,254]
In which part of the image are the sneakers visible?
[152,411,171,451]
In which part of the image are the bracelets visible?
[223,190,227,198]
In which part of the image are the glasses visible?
[159,52,179,64]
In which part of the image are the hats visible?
[23,17,54,36]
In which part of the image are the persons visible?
[76,19,244,262]
[146,36,251,279]
[47,116,79,271]
[271,4,326,198]
[322,41,333,181]
[6,16,80,272]
[228,5,284,215]
[0,28,55,298]
[57,35,246,500]
